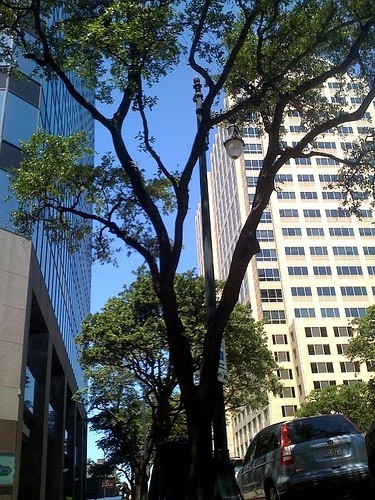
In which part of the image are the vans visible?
[237,413,371,500]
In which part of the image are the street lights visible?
[193,74,246,499]
